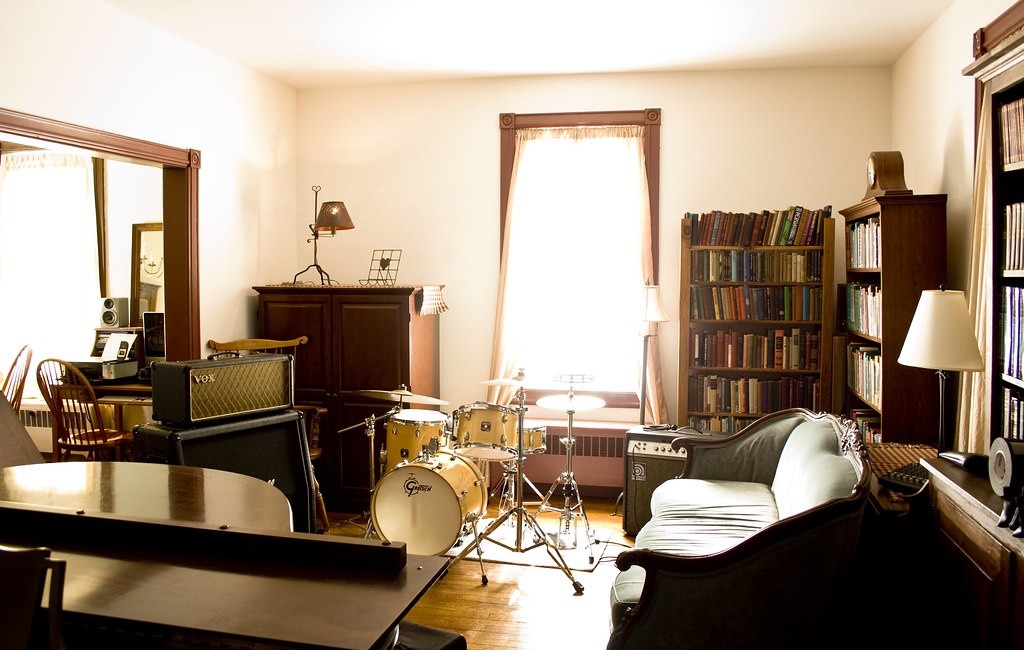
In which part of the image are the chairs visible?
[36,358,135,463]
[209,336,329,531]
[2,344,32,416]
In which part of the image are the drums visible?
[370,449,488,557]
[383,408,449,475]
[517,419,547,455]
[456,402,519,462]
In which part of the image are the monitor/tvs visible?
[143,312,166,366]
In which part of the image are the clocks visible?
[861,151,913,201]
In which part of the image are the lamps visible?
[897,285,984,458]
[139,247,164,279]
[287,184,354,285]
[611,278,669,516]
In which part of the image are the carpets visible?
[443,518,609,571]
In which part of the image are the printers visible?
[58,340,139,386]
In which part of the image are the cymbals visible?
[535,393,607,412]
[352,387,450,407]
[479,378,549,388]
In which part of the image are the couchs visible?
[605,407,872,650]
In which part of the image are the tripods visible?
[439,386,600,592]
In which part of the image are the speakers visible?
[622,424,734,536]
[132,351,317,535]
[100,297,130,328]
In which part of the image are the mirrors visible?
[130,223,164,327]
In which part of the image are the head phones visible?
[137,366,152,384]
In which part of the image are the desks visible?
[0,462,466,650]
[49,384,153,461]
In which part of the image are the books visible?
[689,249,823,281]
[1000,384,1024,440]
[689,373,821,413]
[844,281,881,339]
[689,325,821,370]
[690,286,822,321]
[1003,201,1024,277]
[843,218,881,269]
[999,98,1024,172]
[688,416,756,434]
[685,204,832,245]
[1002,284,1024,387]
[849,406,881,443]
[847,342,881,409]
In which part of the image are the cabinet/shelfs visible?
[254,285,446,493]
[677,218,835,425]
[838,193,947,445]
